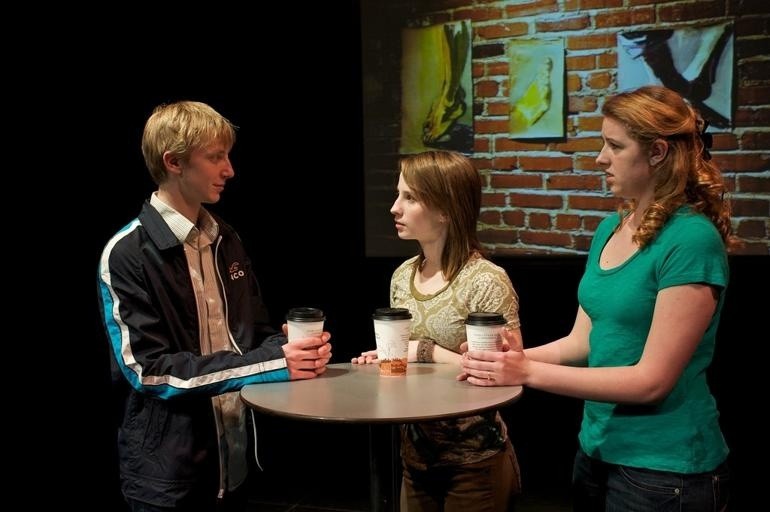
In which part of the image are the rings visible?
[487,373,492,380]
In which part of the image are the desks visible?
[239,360,524,512]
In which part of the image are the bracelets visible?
[417,339,436,364]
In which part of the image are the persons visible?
[457,87,745,511]
[422,24,468,144]
[95,101,332,511]
[510,57,552,136]
[351,152,522,512]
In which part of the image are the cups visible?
[285,307,326,344]
[464,312,508,352]
[372,307,413,380]
